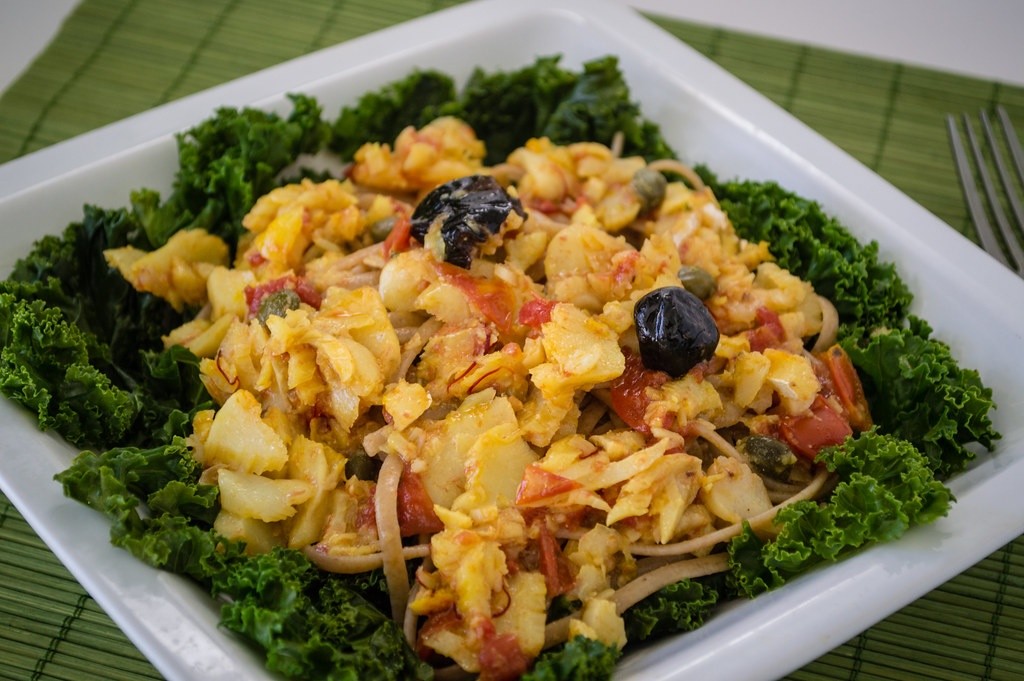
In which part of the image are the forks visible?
[944,103,1024,280]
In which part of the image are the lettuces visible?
[0,50,1004,681]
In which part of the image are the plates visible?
[0,0,1024,681]
